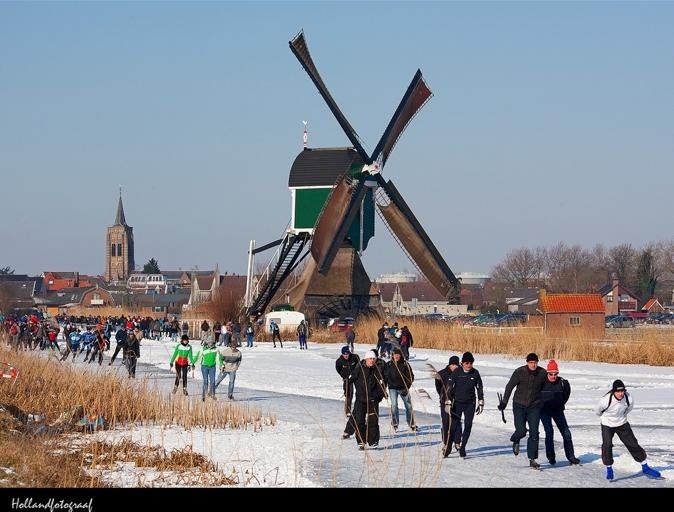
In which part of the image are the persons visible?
[336,322,420,450]
[597,380,661,480]
[443,352,484,455]
[498,353,548,468]
[0,312,307,401]
[435,355,462,451]
[541,359,581,464]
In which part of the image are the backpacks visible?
[247,327,253,334]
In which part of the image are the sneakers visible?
[342,413,418,449]
[171,386,236,402]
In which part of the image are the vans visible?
[0,306,43,326]
[325,316,355,335]
[415,312,528,328]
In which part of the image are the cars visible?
[605,310,674,329]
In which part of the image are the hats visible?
[462,352,474,362]
[449,356,459,365]
[206,335,215,343]
[341,345,350,353]
[526,352,539,362]
[613,379,627,392]
[181,334,189,340]
[363,351,377,359]
[546,359,559,373]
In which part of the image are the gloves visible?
[475,400,485,415]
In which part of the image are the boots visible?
[459,446,467,457]
[641,463,660,478]
[606,466,614,481]
[529,459,540,467]
[512,442,520,455]
[568,456,581,464]
